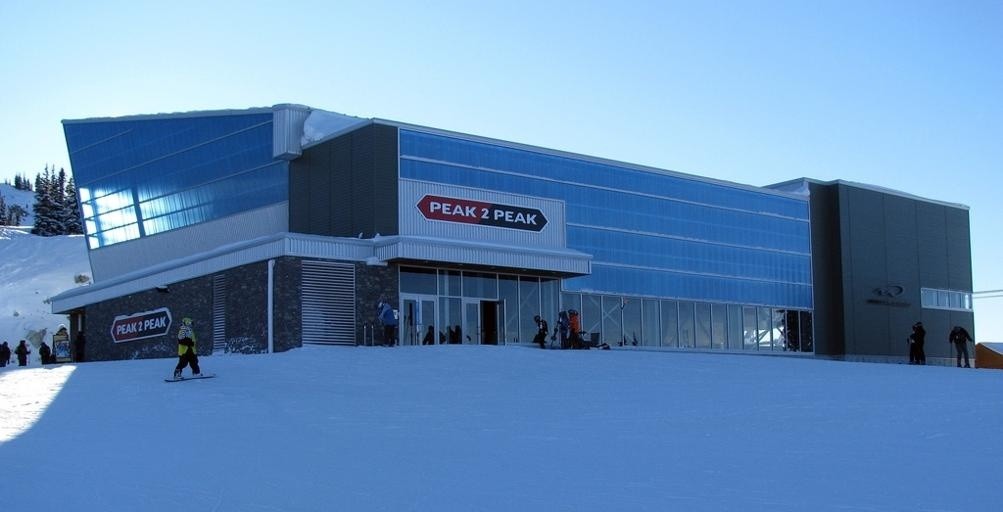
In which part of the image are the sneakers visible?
[174,374,184,379]
[193,373,203,376]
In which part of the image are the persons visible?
[568,309,579,338]
[950,322,973,368]
[912,321,926,365]
[74,330,85,363]
[39,341,55,363]
[534,314,548,349]
[558,310,569,349]
[173,317,203,380]
[15,340,31,367]
[423,324,462,345]
[908,324,917,364]
[0,341,10,368]
[378,300,396,347]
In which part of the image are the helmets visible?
[183,318,193,325]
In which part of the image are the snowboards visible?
[165,374,216,382]
